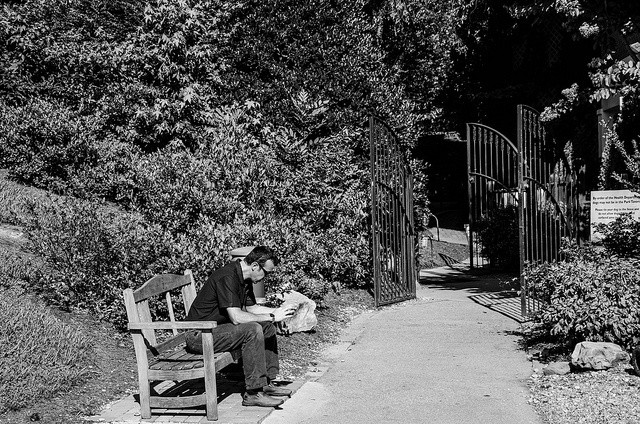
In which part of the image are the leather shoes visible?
[262,381,292,395]
[242,391,284,406]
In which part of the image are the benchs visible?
[123,268,242,421]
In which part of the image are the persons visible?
[186,246,299,407]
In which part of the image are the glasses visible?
[258,263,269,276]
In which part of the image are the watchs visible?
[268,313,276,323]
